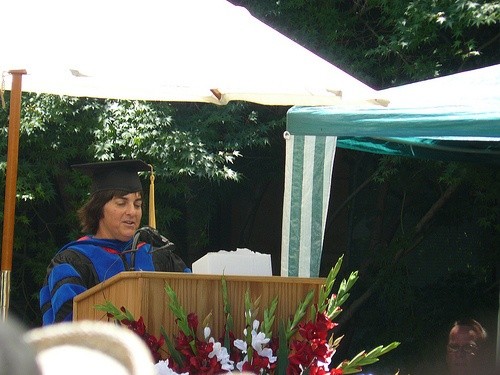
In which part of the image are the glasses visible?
[446,343,478,352]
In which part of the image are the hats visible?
[69,158,156,230]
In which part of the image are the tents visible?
[280,62,499,276]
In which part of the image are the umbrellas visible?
[3,2,371,318]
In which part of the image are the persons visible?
[446,317,489,375]
[41,159,191,325]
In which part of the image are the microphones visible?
[142,225,174,254]
